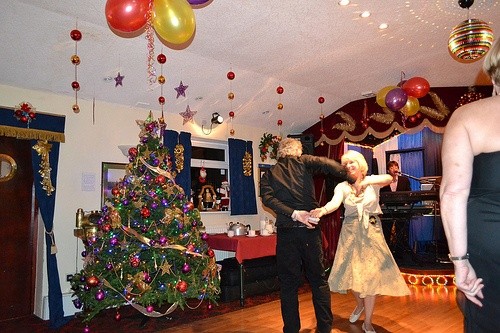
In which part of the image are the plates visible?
[260,233,273,237]
[246,235,258,237]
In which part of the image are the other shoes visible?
[362,322,376,333]
[349,304,364,323]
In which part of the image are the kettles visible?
[227,221,251,236]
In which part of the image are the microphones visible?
[393,169,398,173]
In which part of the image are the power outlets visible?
[201,120,207,126]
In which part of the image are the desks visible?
[204,229,329,308]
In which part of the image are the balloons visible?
[104,0,153,33]
[376,77,430,116]
[152,0,195,44]
[187,0,209,5]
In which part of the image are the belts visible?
[293,224,305,228]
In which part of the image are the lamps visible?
[210,112,224,124]
[447,0,494,64]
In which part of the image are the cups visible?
[228,231,234,237]
[248,230,255,236]
[260,230,268,234]
[266,225,274,234]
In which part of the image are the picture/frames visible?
[100,162,130,210]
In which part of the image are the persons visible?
[260,138,361,333]
[310,149,410,333]
[379,160,412,260]
[439,36,500,333]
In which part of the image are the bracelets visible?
[323,206,328,214]
[448,253,469,261]
